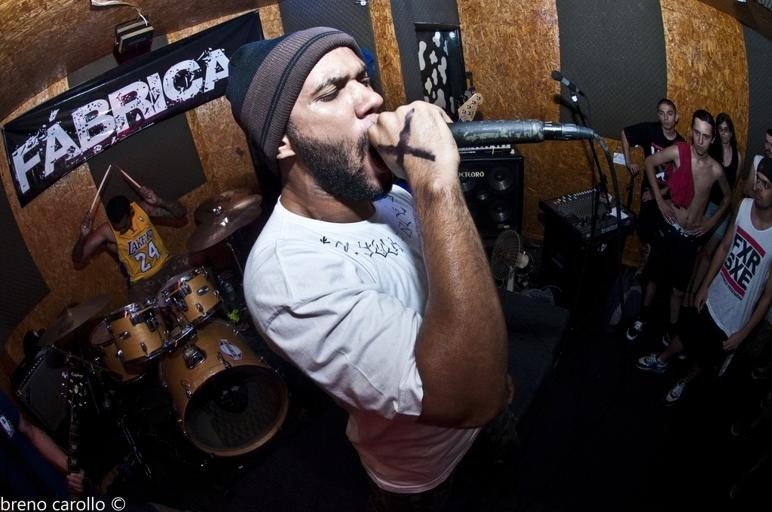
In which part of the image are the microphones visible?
[550,69,589,105]
[447,117,598,156]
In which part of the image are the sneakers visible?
[626,318,691,403]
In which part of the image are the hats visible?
[226,26,366,173]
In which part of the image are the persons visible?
[679,111,743,307]
[740,125,772,200]
[0,391,92,512]
[624,107,734,348]
[634,154,771,404]
[619,98,687,277]
[224,24,530,508]
[71,184,189,296]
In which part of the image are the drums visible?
[156,318,288,457]
[158,265,223,327]
[106,298,174,366]
[91,325,146,384]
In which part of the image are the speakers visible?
[7,349,68,436]
[448,154,524,254]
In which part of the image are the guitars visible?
[454,91,485,120]
[56,355,94,509]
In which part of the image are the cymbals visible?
[37,294,112,350]
[186,188,262,253]
